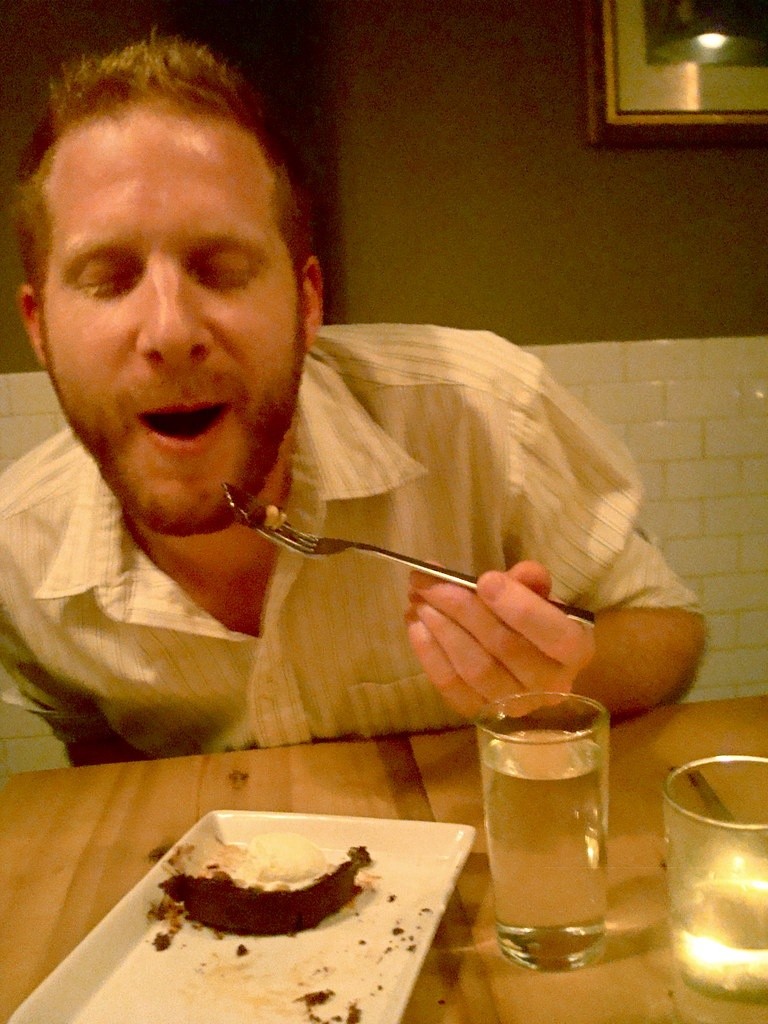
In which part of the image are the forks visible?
[221,482,595,630]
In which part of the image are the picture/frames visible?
[583,0,768,150]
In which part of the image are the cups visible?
[473,692,607,971]
[661,754,768,1024]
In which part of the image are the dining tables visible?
[0,693,768,1024]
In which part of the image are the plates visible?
[5,810,478,1023]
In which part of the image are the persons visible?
[0,23,709,771]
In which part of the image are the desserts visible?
[156,831,371,939]
